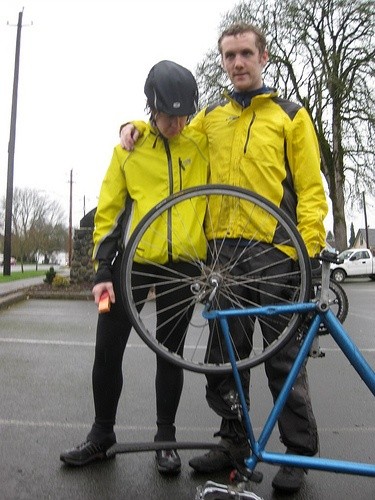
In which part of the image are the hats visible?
[144,60,198,115]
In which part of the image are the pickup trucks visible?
[330,248,375,283]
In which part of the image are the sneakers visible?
[59,430,117,466]
[154,427,181,473]
[272,450,309,490]
[188,442,251,471]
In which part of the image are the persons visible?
[59,59,209,475]
[117,22,330,492]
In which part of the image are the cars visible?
[10,256,17,265]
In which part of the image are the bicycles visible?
[106,184,375,500]
